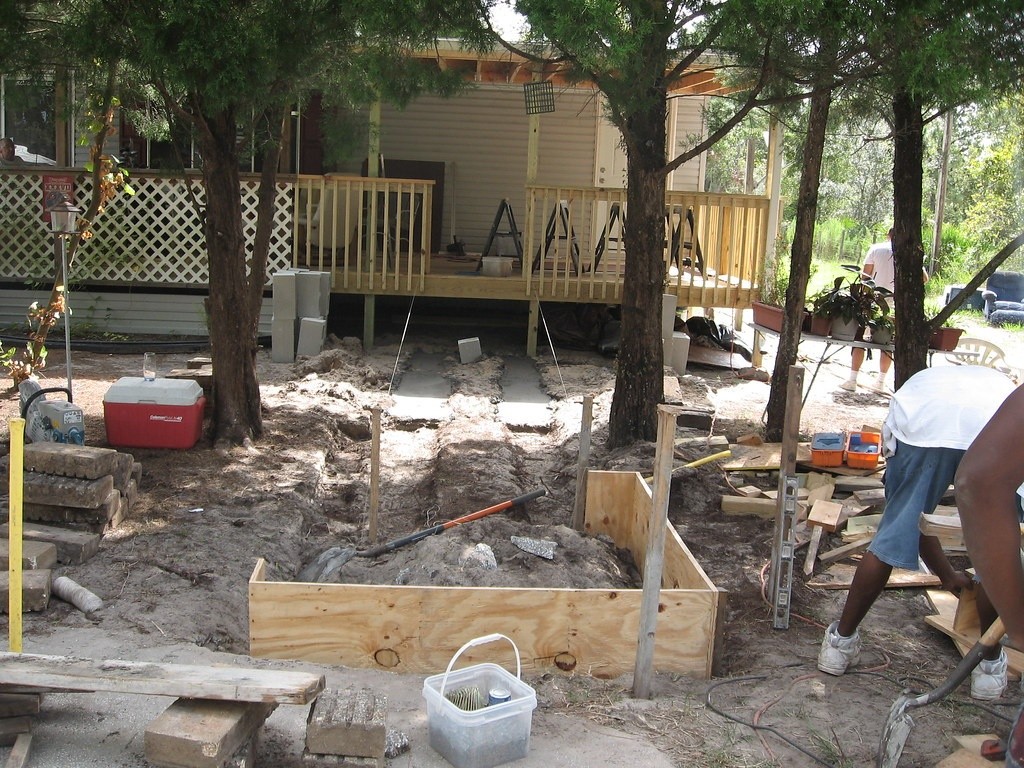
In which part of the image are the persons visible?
[953,385,1024,768]
[0,138,26,167]
[817,366,1024,700]
[841,226,929,393]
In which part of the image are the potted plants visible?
[807,276,832,337]
[814,264,894,342]
[869,312,896,345]
[924,302,968,351]
[751,232,809,332]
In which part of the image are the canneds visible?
[489,687,512,706]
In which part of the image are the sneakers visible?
[839,379,856,391]
[818,620,862,676]
[971,647,1008,700]
[871,383,883,394]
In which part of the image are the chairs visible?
[357,192,420,271]
[945,337,1024,386]
[292,171,363,267]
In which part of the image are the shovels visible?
[298,489,546,584]
[877,616,1008,768]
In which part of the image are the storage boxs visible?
[102,377,206,449]
[845,431,882,470]
[495,231,524,257]
[421,662,538,768]
[810,431,846,467]
[482,256,513,278]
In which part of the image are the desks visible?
[748,322,980,428]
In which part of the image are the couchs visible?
[982,271,1024,329]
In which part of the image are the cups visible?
[143,351,157,382]
